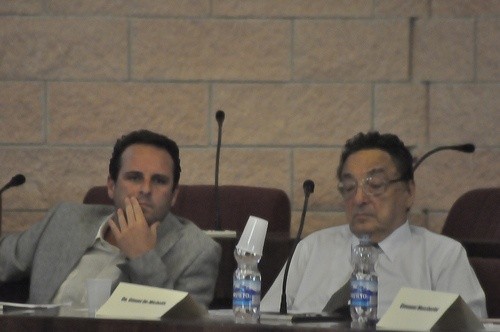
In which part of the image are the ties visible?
[322,264,371,314]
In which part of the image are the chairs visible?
[81,185,500,319]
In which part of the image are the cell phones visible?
[293,313,344,322]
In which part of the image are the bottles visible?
[233,251,262,330]
[349,235,378,332]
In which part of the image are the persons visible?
[260,130,488,320]
[0,129,222,310]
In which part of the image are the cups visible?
[236,217,268,259]
[86,279,112,317]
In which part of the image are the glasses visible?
[338,175,402,197]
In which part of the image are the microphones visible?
[0,174,26,193]
[413,144,475,170]
[280,179,314,314]
[214,110,224,229]
